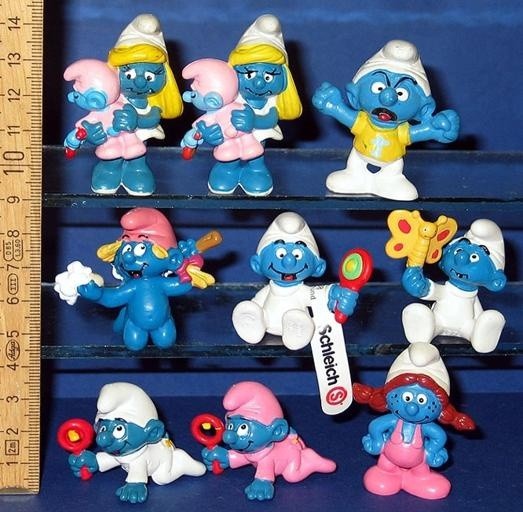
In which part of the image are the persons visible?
[44,12,510,509]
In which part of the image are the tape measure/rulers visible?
[0,0,44,495]
